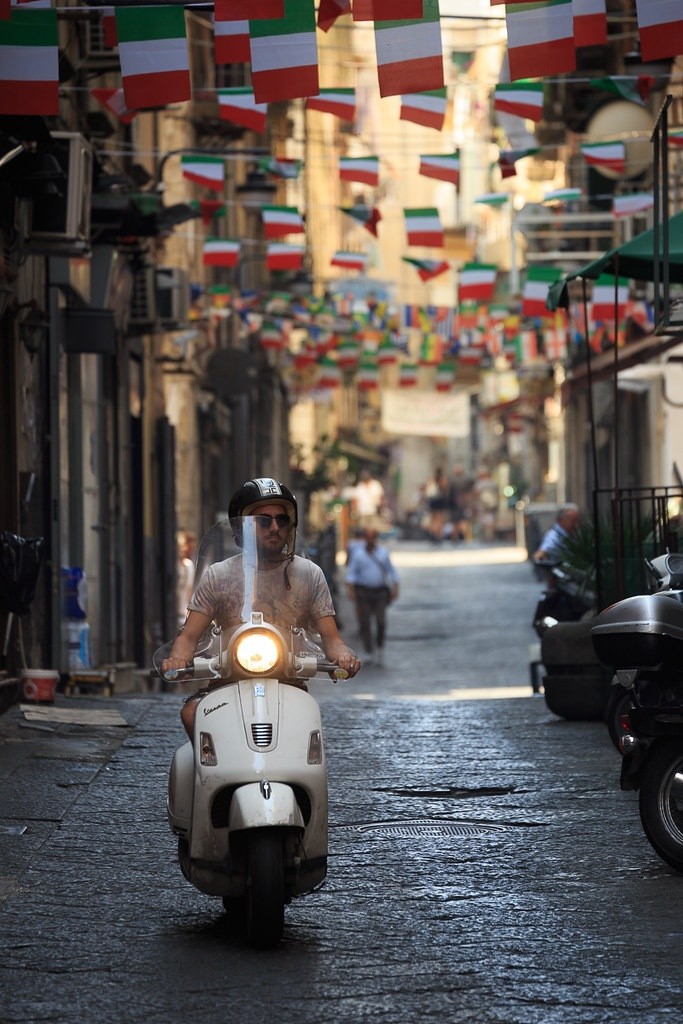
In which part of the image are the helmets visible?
[228,477,300,556]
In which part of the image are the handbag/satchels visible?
[382,574,395,598]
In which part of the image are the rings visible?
[350,664,355,669]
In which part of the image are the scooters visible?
[149,515,361,950]
[588,589,683,874]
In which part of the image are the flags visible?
[0,0,683,392]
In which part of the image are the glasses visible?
[256,513,292,528]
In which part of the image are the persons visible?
[161,478,361,749]
[344,528,399,663]
[327,468,385,528]
[532,501,579,590]
[419,463,499,548]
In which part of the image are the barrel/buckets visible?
[20,668,60,706]
